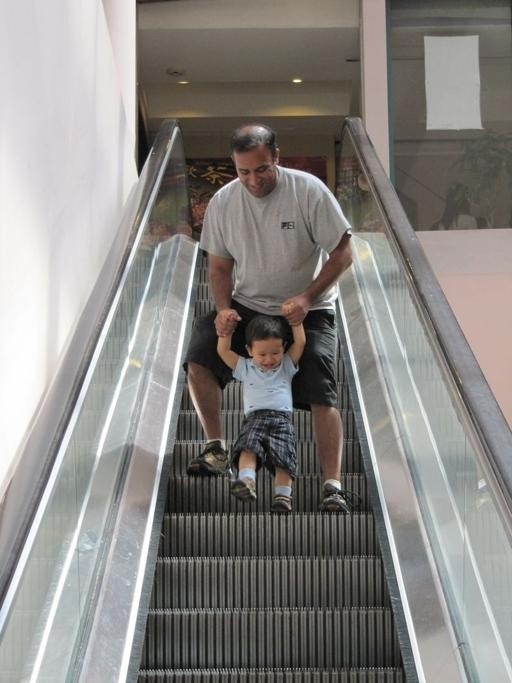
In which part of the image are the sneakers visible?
[271,493,293,512]
[319,483,351,512]
[187,440,232,474]
[231,476,257,502]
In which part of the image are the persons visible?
[449,183,478,229]
[215,302,307,511]
[182,123,356,513]
